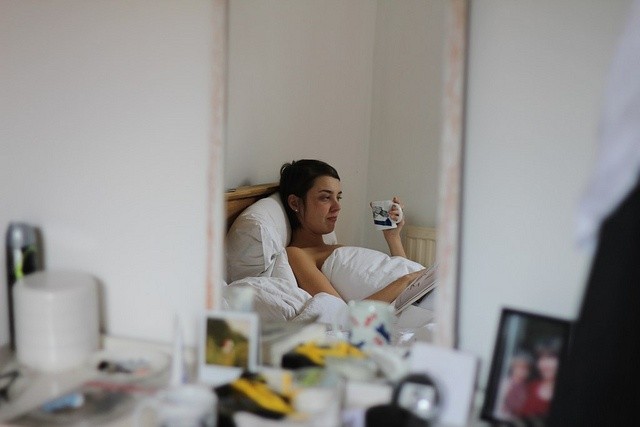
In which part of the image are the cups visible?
[370,200,405,231]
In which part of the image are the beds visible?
[223,183,439,317]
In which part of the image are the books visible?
[396,264,437,313]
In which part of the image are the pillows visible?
[223,190,339,281]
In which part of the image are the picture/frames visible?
[479,308,573,427]
[195,309,261,386]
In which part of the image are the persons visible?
[524,343,559,419]
[278,159,435,308]
[494,350,532,424]
